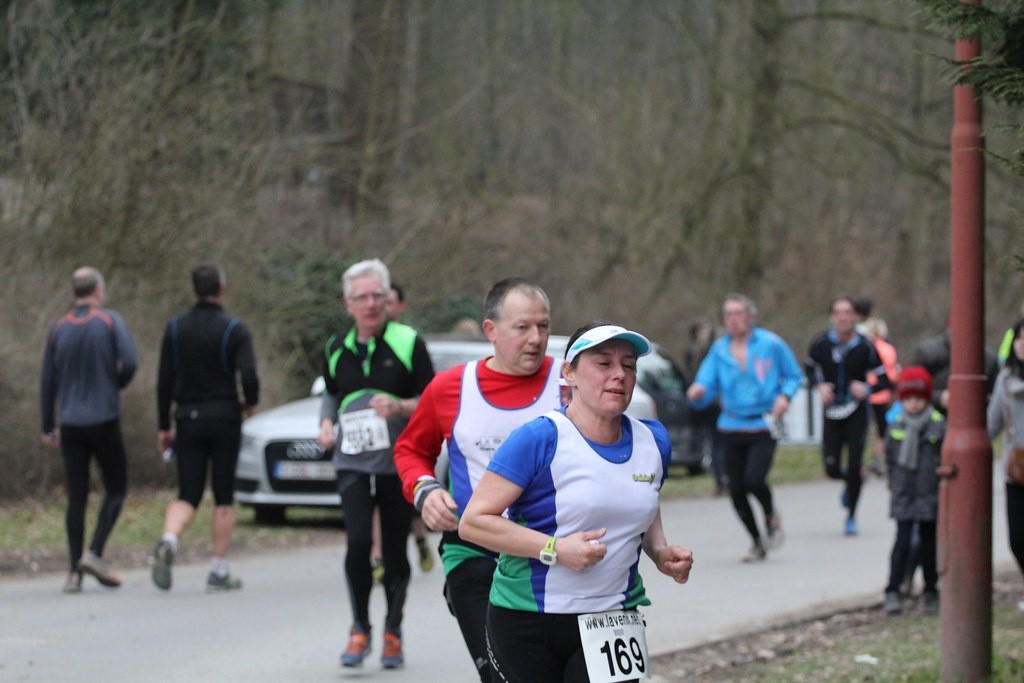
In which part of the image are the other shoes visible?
[764,514,786,549]
[884,591,904,614]
[741,545,767,564]
[76,549,123,586]
[151,541,173,590]
[845,517,858,537]
[841,488,850,506]
[920,592,940,615]
[206,571,242,595]
[61,571,83,596]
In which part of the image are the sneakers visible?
[340,626,373,666]
[380,631,406,668]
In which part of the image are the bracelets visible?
[414,484,440,510]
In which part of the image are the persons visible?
[987,316,1024,611]
[39,267,137,593]
[683,321,729,496]
[318,259,436,668]
[685,292,804,560]
[152,262,259,589]
[450,317,483,336]
[457,322,694,683]
[375,282,434,583]
[917,311,1000,421]
[883,365,945,616]
[862,315,903,476]
[854,297,874,334]
[805,297,888,536]
[394,276,572,683]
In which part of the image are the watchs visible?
[539,536,558,565]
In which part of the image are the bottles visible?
[763,411,786,440]
[162,446,177,465]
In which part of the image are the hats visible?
[897,365,934,402]
[565,324,654,366]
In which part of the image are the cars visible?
[629,346,720,475]
[235,338,662,532]
